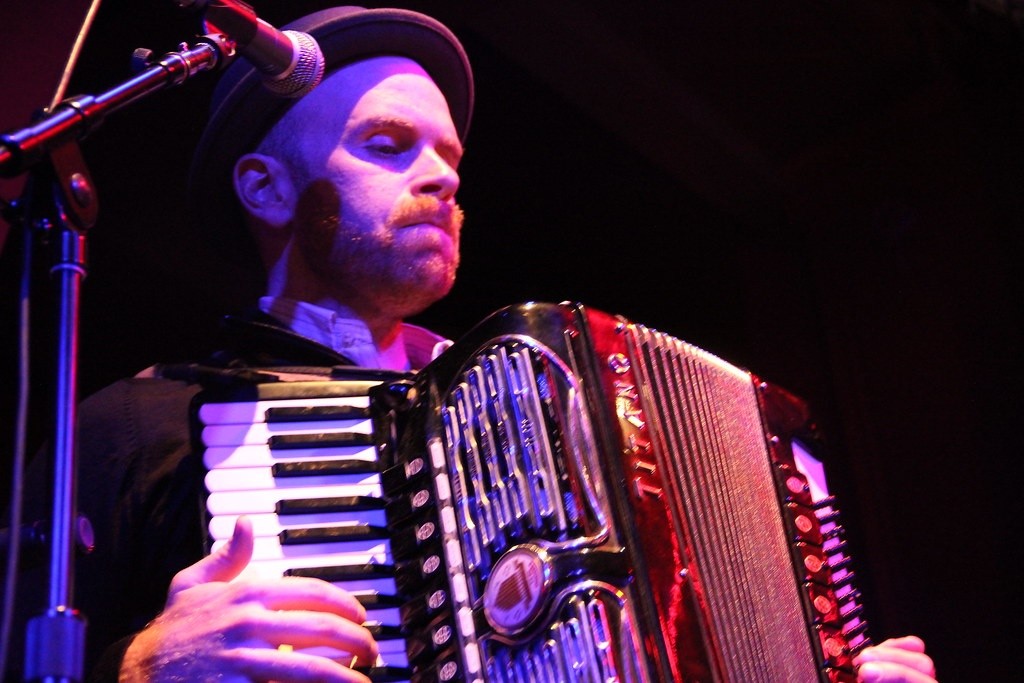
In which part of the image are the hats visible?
[188,5,472,202]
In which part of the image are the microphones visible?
[181,0,325,100]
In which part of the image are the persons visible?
[18,2,939,681]
